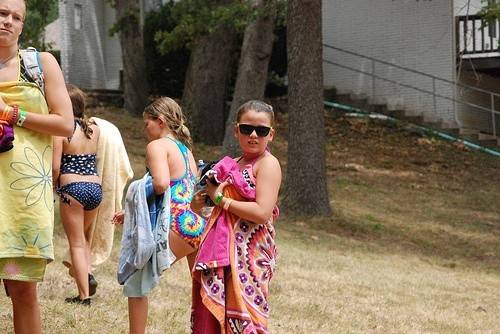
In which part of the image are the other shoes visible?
[65,296,91,306]
[89,275,97,296]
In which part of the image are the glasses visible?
[237,123,272,137]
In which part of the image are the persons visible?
[190,100,283,334]
[0,0,75,334]
[110,97,207,334]
[52,83,103,308]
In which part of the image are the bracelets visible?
[216,193,224,207]
[16,109,27,126]
[1,104,11,119]
[9,105,18,124]
[223,198,233,211]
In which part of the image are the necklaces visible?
[0,50,18,69]
